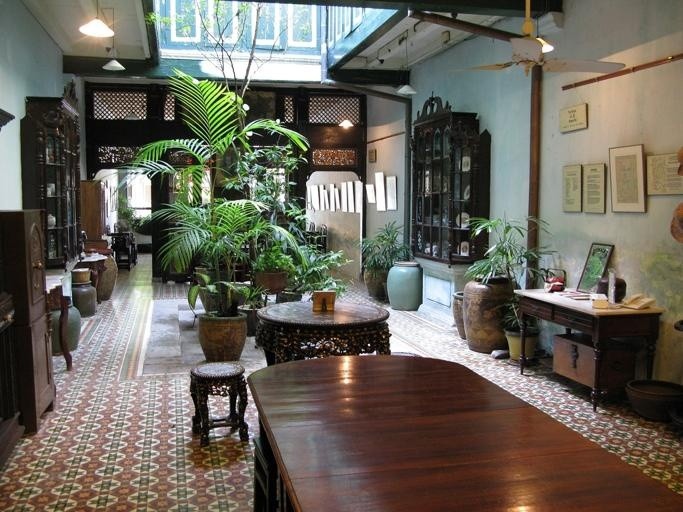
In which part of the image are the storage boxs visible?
[552,333,635,391]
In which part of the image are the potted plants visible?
[93,66,311,362]
[463,210,559,362]
[357,221,414,303]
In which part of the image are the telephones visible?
[622,293,655,310]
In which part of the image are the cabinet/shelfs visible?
[19,78,81,270]
[410,91,481,265]
[0,207,58,434]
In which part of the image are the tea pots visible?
[540,268,566,292]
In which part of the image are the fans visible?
[465,0,625,78]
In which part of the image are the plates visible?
[458,157,470,172]
[454,213,469,228]
[463,185,470,200]
[457,242,474,257]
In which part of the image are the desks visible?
[248,356,682,511]
[512,289,666,413]
[256,301,391,368]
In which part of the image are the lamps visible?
[534,17,554,54]
[395,37,417,96]
[77,0,125,72]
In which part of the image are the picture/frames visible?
[576,242,615,294]
[607,144,647,214]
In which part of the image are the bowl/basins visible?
[624,378,681,420]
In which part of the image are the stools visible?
[188,362,249,447]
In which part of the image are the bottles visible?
[40,136,57,262]
